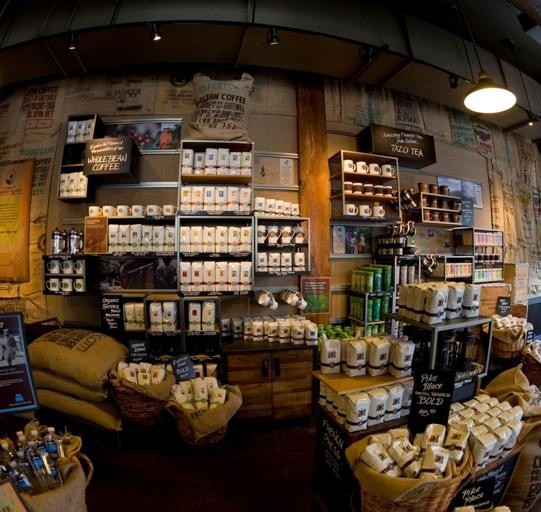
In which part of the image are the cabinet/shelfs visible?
[312,309,495,511]
[225,340,314,420]
[44,110,312,335]
[410,190,463,228]
[331,149,403,228]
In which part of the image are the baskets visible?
[492,325,541,389]
[360,451,473,512]
[109,383,228,447]
[18,453,94,512]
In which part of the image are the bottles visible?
[0,427,66,497]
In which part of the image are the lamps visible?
[463,2,516,114]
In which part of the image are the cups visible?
[344,181,394,198]
[73,278,85,292]
[51,227,84,255]
[343,160,394,177]
[281,288,308,310]
[59,279,73,292]
[73,259,85,275]
[47,260,60,274]
[60,259,74,274]
[46,278,60,292]
[88,204,177,217]
[258,292,279,310]
[257,225,307,244]
[417,182,450,196]
[423,209,451,222]
[345,204,385,217]
